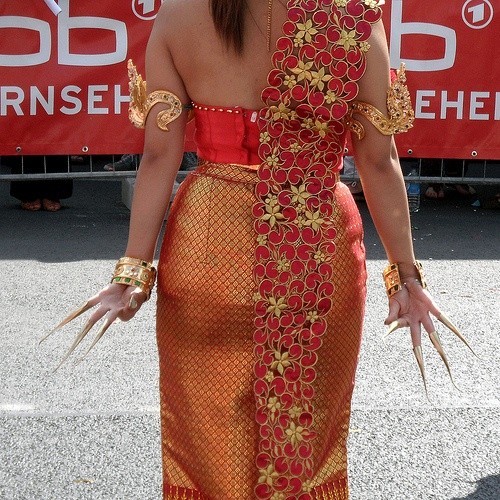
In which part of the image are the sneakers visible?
[104,154,139,170]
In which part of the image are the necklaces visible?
[266,1,272,51]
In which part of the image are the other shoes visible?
[21,199,61,211]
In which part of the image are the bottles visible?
[407,170,420,212]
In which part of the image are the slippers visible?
[427,183,444,198]
[455,183,476,196]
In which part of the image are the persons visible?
[38,0,479,500]
[10,153,476,210]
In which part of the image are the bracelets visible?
[111,257,156,302]
[382,263,427,298]
